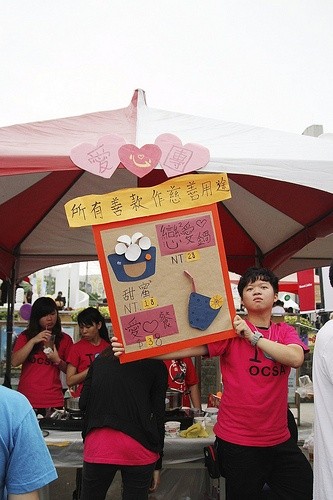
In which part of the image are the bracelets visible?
[29,340,36,345]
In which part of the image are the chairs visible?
[287,368,301,426]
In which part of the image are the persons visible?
[272,299,321,331]
[312,263,333,500]
[55,292,66,306]
[0,384,58,500]
[66,307,113,398]
[11,297,73,416]
[78,345,168,500]
[164,356,202,418]
[110,267,314,500]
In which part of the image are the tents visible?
[0,89,333,387]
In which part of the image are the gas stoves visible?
[165,409,193,429]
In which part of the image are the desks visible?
[37,417,226,500]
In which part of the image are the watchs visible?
[250,331,264,346]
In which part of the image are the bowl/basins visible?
[165,421,181,439]
[194,417,211,424]
[206,408,218,417]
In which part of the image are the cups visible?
[43,334,56,351]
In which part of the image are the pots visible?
[165,388,190,413]
[64,397,80,414]
